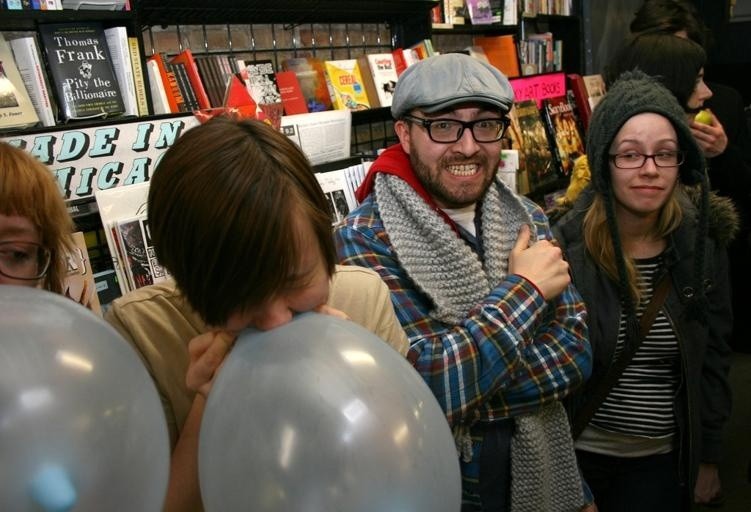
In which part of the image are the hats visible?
[587,70,709,188]
[391,53,513,120]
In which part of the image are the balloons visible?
[197,316,464,511]
[2,286,174,510]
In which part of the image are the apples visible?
[695,111,712,125]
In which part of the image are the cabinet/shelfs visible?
[0,0,592,315]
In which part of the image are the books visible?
[1,1,132,10]
[495,73,607,225]
[279,110,373,233]
[431,0,573,81]
[0,21,149,131]
[146,38,436,114]
[48,180,176,313]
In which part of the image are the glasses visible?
[404,114,510,144]
[608,150,688,169]
[0,240,52,280]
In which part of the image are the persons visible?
[629,31,745,190]
[544,68,738,508]
[332,51,598,509]
[632,1,702,38]
[106,117,413,509]
